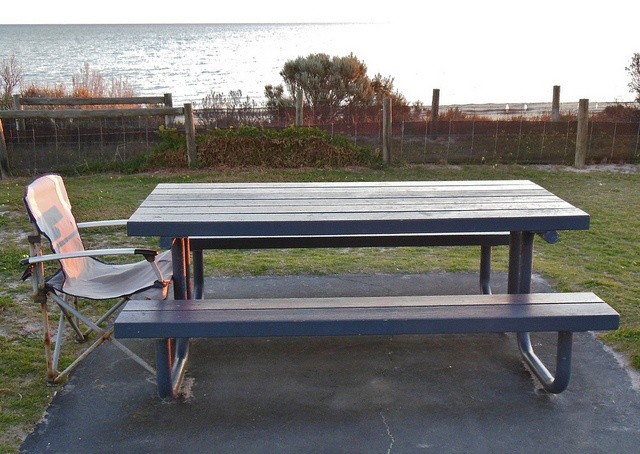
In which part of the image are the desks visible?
[127,179,590,237]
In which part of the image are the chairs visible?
[20,174,173,387]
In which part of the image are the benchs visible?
[161,232,510,251]
[114,291,623,338]
